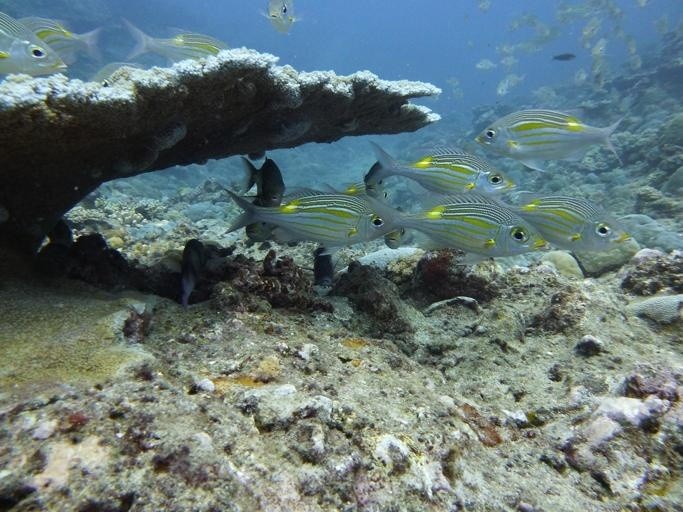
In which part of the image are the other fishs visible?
[221,140,548,257]
